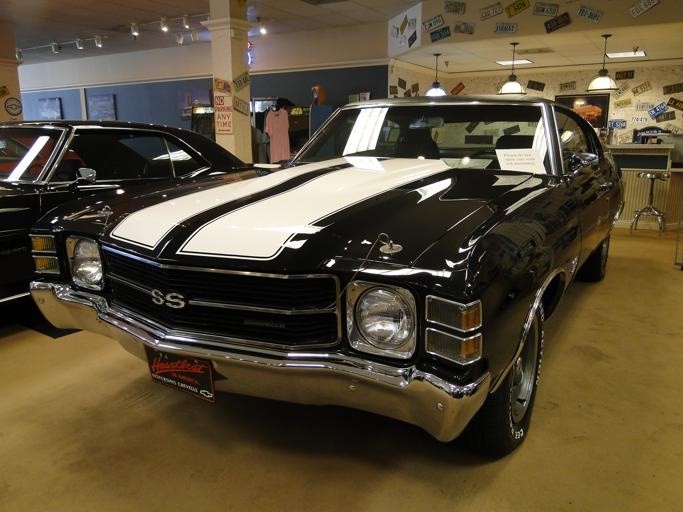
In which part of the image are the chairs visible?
[495,134,547,174]
[388,132,440,159]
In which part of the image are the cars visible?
[29,94,624,461]
[0,120,252,330]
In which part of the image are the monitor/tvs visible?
[555,93,610,130]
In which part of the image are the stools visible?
[626,171,671,234]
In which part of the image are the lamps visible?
[496,42,526,94]
[424,53,446,97]
[14,35,104,59]
[131,13,266,37]
[584,32,618,93]
